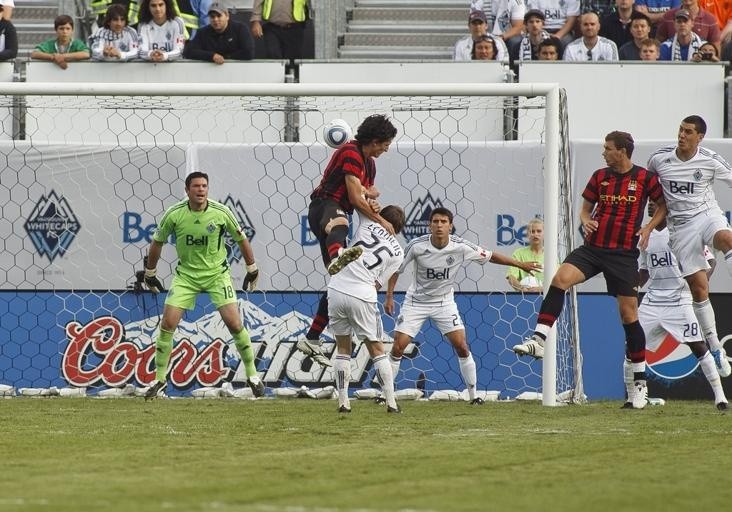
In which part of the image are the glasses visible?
[474,37,493,42]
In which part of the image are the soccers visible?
[322,116,354,148]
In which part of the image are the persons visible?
[252,0,314,58]
[619,17,663,60]
[512,9,563,60]
[471,35,499,60]
[455,10,509,62]
[0,5,20,65]
[378,208,541,405]
[697,0,731,54]
[512,130,666,409]
[143,170,269,399]
[660,10,710,65]
[636,0,683,41]
[327,176,403,415]
[640,37,660,60]
[692,42,718,61]
[472,0,527,46]
[193,1,255,64]
[27,16,91,70]
[298,113,397,368]
[602,0,655,44]
[136,1,188,61]
[528,1,579,46]
[649,0,719,53]
[621,189,729,412]
[563,11,618,62]
[535,38,561,59]
[644,116,732,379]
[87,7,140,61]
[507,217,557,294]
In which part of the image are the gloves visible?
[243,262,259,293]
[144,266,163,294]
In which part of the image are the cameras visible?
[701,53,714,60]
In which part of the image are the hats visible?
[674,9,694,22]
[469,10,488,25]
[206,1,228,14]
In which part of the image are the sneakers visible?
[296,335,333,368]
[326,246,363,276]
[337,405,352,419]
[512,339,545,360]
[246,376,265,398]
[711,379,728,411]
[143,381,168,399]
[373,396,386,407]
[468,398,485,406]
[632,385,649,409]
[386,404,406,417]
[617,402,644,411]
[710,346,731,377]
